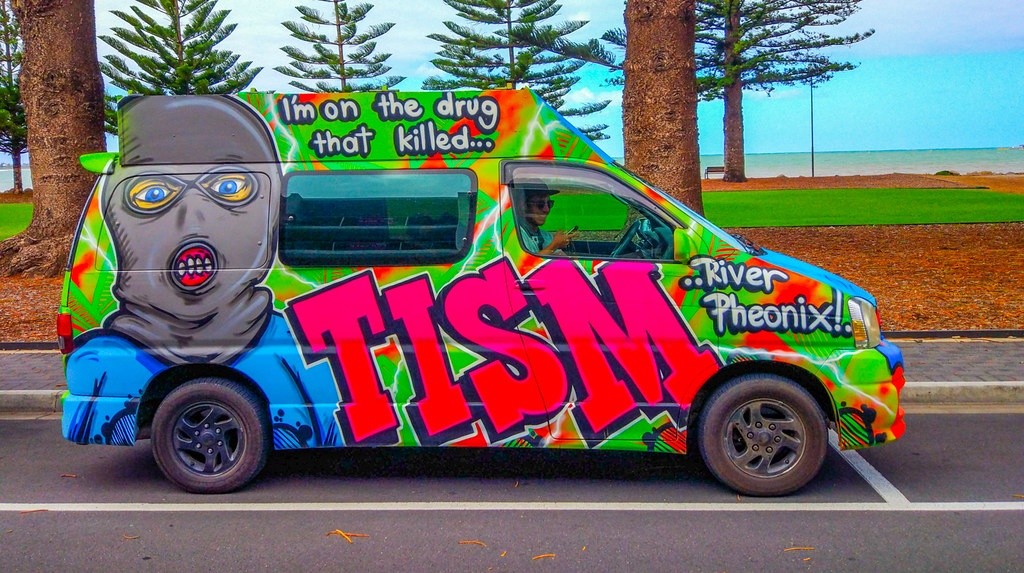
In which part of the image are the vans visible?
[56,83,909,499]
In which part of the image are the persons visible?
[515,178,577,256]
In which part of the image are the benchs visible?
[705,167,725,179]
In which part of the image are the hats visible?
[512,178,560,195]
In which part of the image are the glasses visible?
[528,200,555,208]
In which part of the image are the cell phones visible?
[566,225,578,235]
